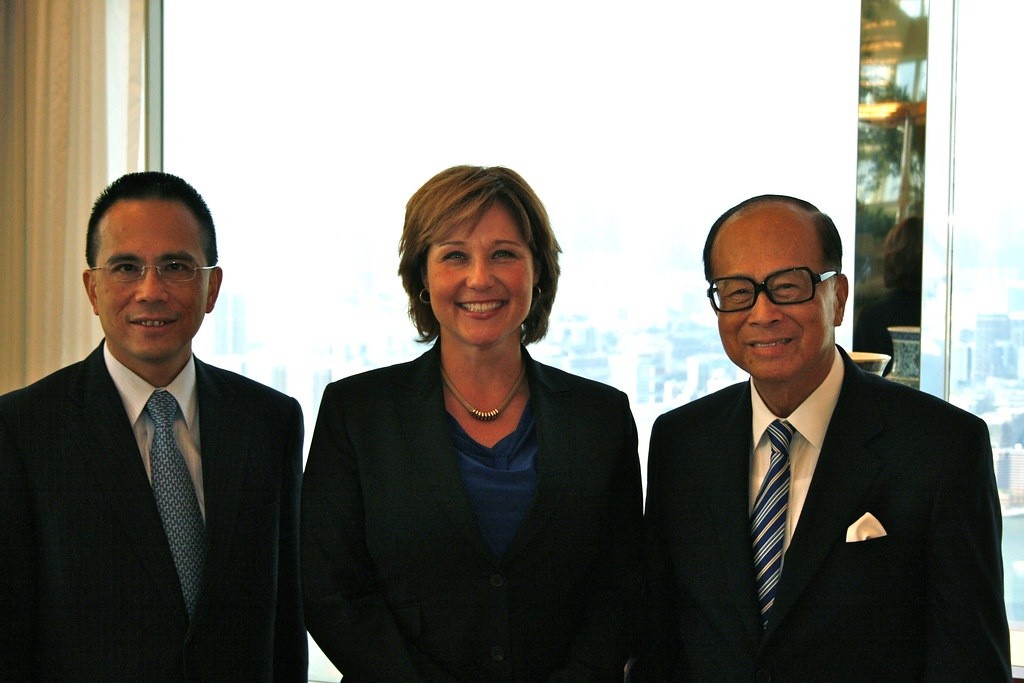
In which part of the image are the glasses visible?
[91,257,217,282]
[707,267,838,313]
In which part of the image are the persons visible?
[0,171,313,683]
[286,163,642,683]
[641,195,1013,681]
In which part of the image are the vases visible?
[884,325,921,392]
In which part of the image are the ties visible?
[751,420,798,629]
[143,390,208,623]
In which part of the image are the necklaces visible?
[440,361,528,421]
